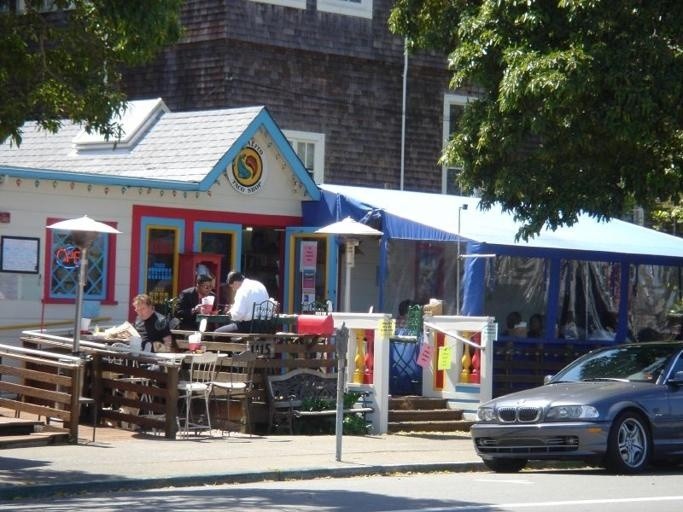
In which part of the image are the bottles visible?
[147,263,171,280]
[148,287,171,304]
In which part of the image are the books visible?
[95,322,140,343]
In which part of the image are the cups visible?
[188,343,200,352]
[195,349,202,353]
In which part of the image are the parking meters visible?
[332,322,349,463]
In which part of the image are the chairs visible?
[107,342,154,437]
[249,299,281,335]
[175,350,249,441]
[308,301,328,313]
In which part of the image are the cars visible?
[469,340,682,472]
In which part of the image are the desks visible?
[195,313,298,343]
[143,352,230,440]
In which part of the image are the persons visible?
[210,271,270,342]
[500,304,663,352]
[129,294,171,355]
[394,301,415,327]
[173,270,214,332]
